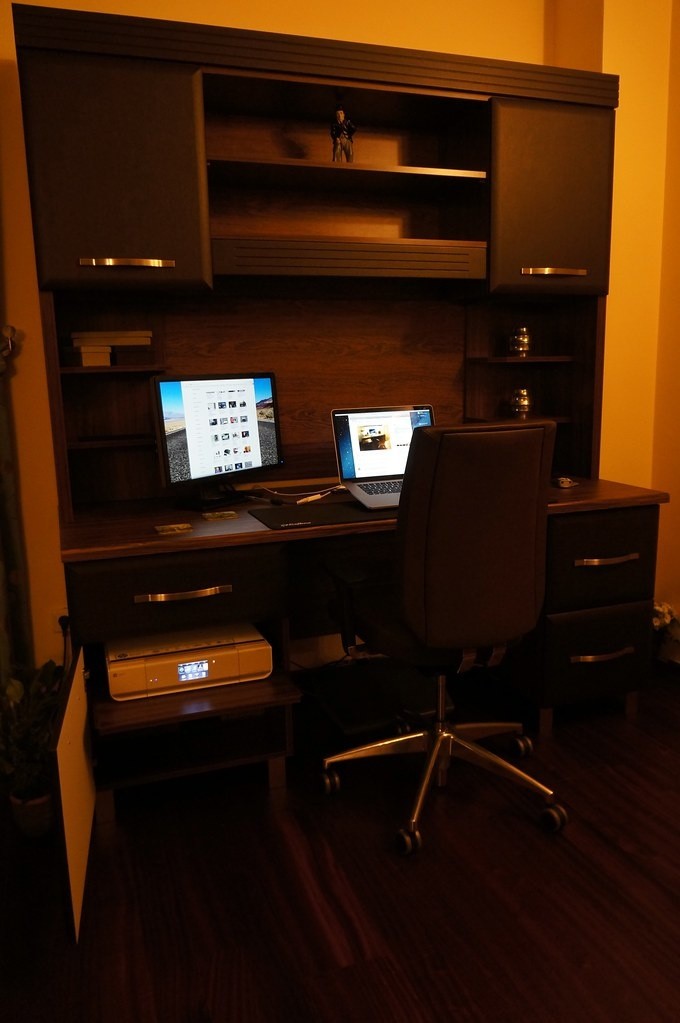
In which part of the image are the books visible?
[69,328,156,367]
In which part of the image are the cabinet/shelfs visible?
[14,2,672,832]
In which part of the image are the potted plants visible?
[2,660,70,835]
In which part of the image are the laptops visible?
[332,405,436,509]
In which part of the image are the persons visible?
[329,106,356,163]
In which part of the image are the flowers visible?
[649,604,680,645]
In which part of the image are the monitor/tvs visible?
[149,372,283,512]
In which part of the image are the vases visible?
[652,645,662,662]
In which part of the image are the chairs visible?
[315,418,568,860]
